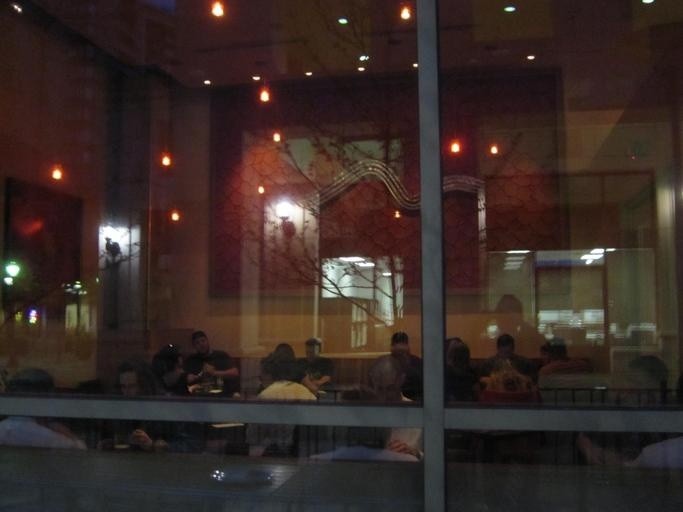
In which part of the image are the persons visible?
[464,294,552,467]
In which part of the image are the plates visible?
[205,465,274,484]
